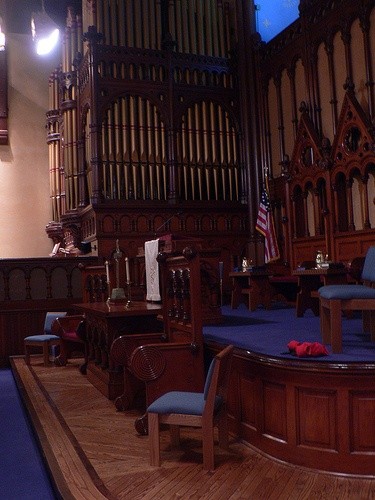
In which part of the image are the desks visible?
[73,301,162,399]
[229,272,272,311]
[291,267,350,318]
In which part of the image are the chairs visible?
[23,312,66,367]
[54,261,114,376]
[111,245,204,434]
[317,247,375,353]
[146,344,234,471]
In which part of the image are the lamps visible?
[31,0,60,59]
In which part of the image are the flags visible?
[255,183,281,263]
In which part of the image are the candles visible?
[106,262,110,282]
[125,258,130,282]
